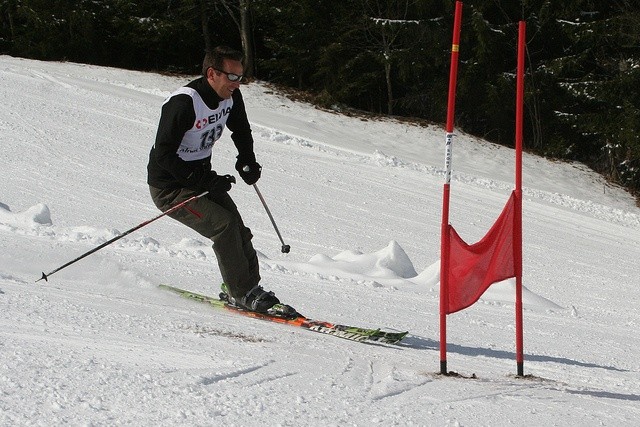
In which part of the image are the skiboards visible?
[158,280,409,347]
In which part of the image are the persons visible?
[147,42,280,317]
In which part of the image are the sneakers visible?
[229,285,279,312]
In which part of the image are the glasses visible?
[212,67,244,83]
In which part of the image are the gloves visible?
[200,170,231,196]
[238,162,261,184]
